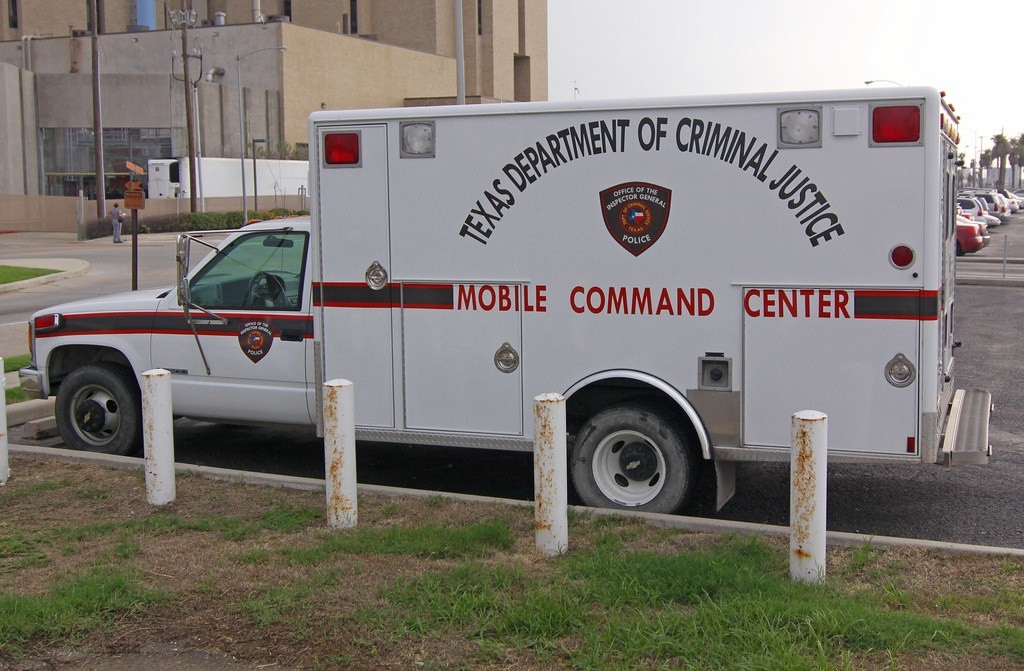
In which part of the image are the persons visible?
[110,203,125,243]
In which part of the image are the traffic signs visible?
[123,161,145,210]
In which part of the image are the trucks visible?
[18,85,995,515]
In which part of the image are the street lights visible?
[236,46,288,226]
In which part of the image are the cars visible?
[952,187,1024,257]
